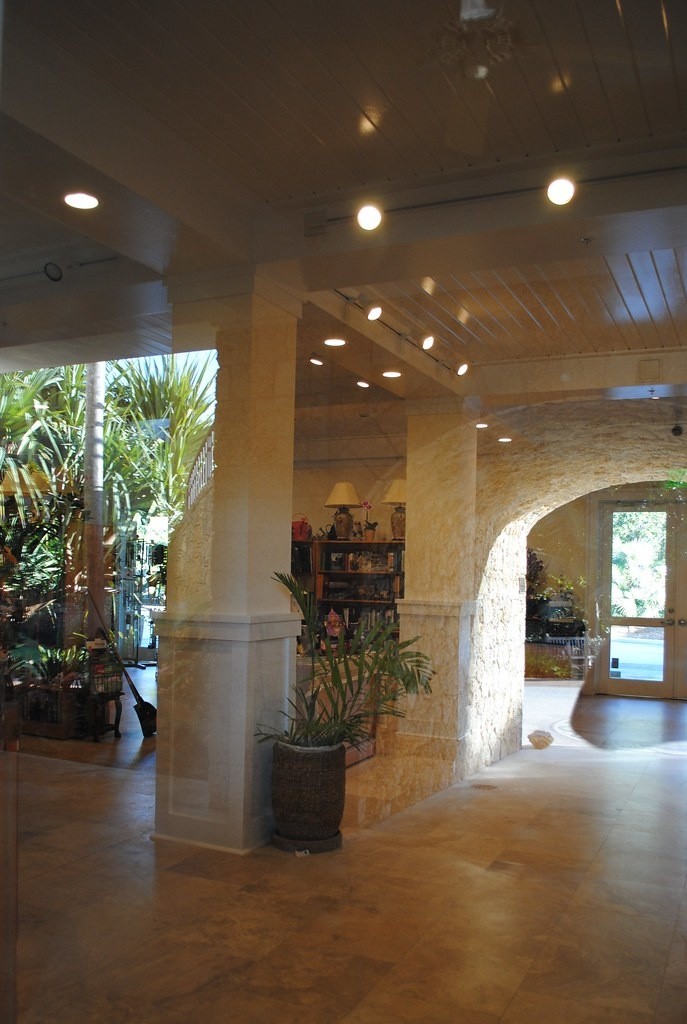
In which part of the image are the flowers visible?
[359,500,378,530]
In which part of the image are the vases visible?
[362,529,375,541]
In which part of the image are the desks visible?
[79,692,125,743]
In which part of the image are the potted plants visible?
[18,646,103,740]
[252,570,439,839]
[526,548,560,592]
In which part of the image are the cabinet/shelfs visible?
[291,541,406,658]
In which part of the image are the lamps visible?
[44,254,79,282]
[438,355,469,377]
[381,479,407,541]
[400,326,434,350]
[324,482,363,540]
[349,293,382,321]
